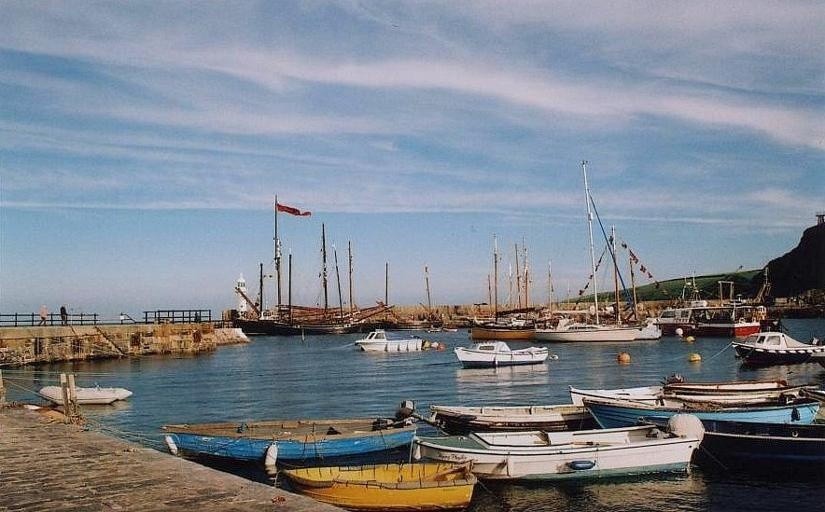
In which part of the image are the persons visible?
[60,304,69,326]
[38,304,48,326]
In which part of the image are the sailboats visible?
[228,194,471,341]
[469,229,643,341]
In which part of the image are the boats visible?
[354,328,424,354]
[640,415,824,477]
[427,396,600,436]
[568,379,818,406]
[38,385,133,404]
[413,422,698,480]
[635,266,785,337]
[582,394,820,430]
[283,463,478,512]
[453,340,549,369]
[732,331,824,365]
[160,415,420,463]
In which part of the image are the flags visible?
[573,236,677,312]
